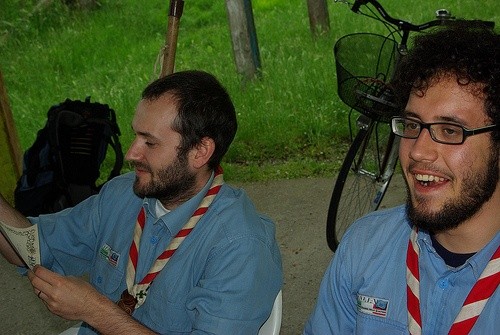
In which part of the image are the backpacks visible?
[14,96,124,218]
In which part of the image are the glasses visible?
[391,116,498,145]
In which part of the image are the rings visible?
[37,290,42,298]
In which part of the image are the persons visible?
[0,69,283,335]
[300,28,500,335]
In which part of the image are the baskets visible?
[334,33,411,122]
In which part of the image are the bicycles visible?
[326,0,496,256]
